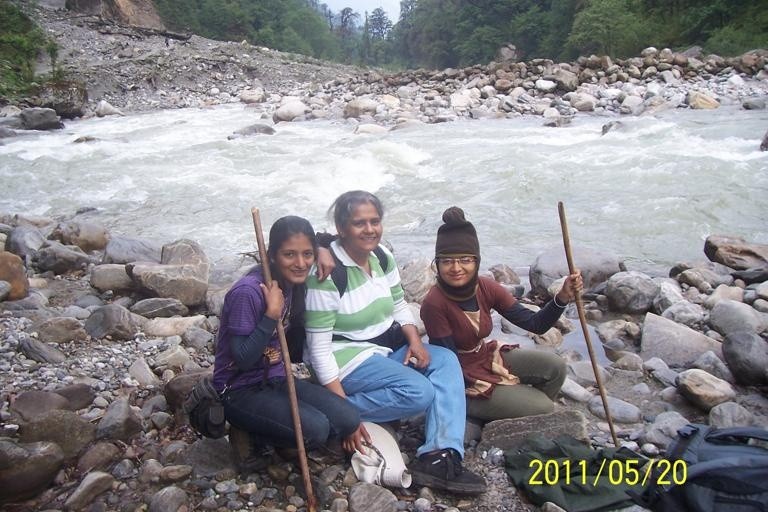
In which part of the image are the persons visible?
[305,188,489,494]
[419,206,586,418]
[211,214,364,505]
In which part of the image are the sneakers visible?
[412,448,488,495]
[228,426,299,472]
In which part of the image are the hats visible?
[349,421,412,488]
[434,206,481,296]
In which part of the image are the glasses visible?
[438,257,473,264]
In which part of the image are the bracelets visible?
[552,288,570,309]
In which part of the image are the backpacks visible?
[644,422,768,511]
